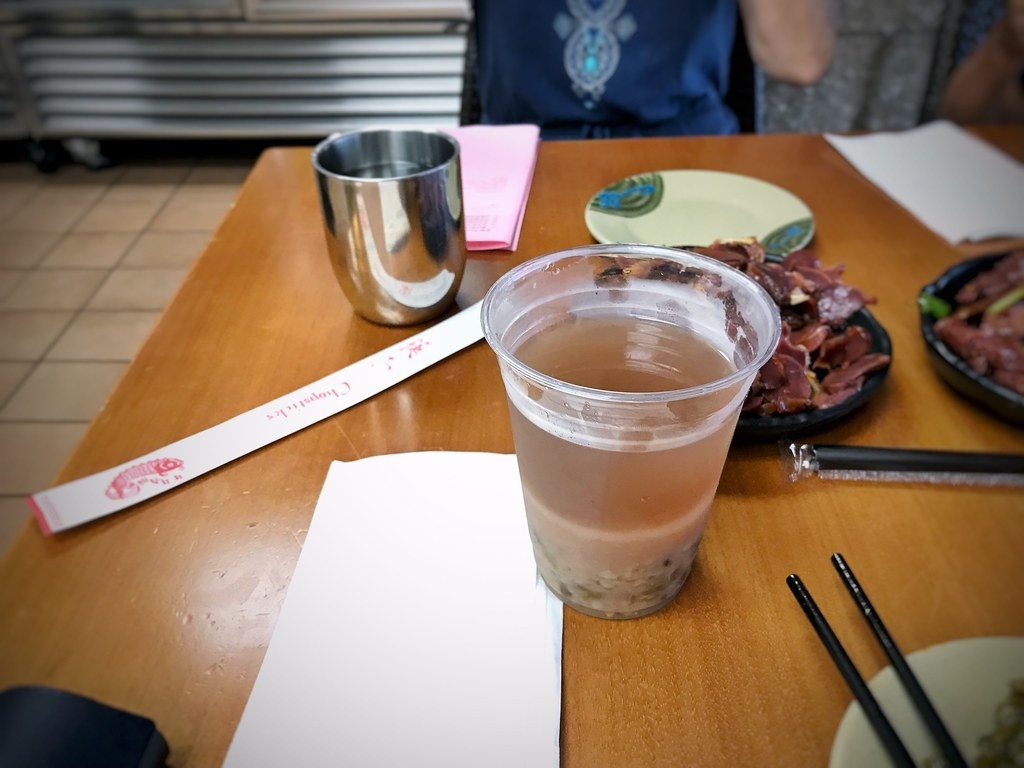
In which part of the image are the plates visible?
[727,304,892,442]
[919,249,1024,434]
[827,634,1024,768]
[583,168,817,266]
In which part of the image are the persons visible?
[460,0,835,141]
[937,0,1024,126]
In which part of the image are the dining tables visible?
[0,122,1024,768]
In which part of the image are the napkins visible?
[436,124,541,252]
[820,118,1024,247]
[220,452,563,768]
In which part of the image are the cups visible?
[478,243,783,620]
[309,126,467,327]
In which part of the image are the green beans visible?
[917,290,949,315]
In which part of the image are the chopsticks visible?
[784,551,970,768]
